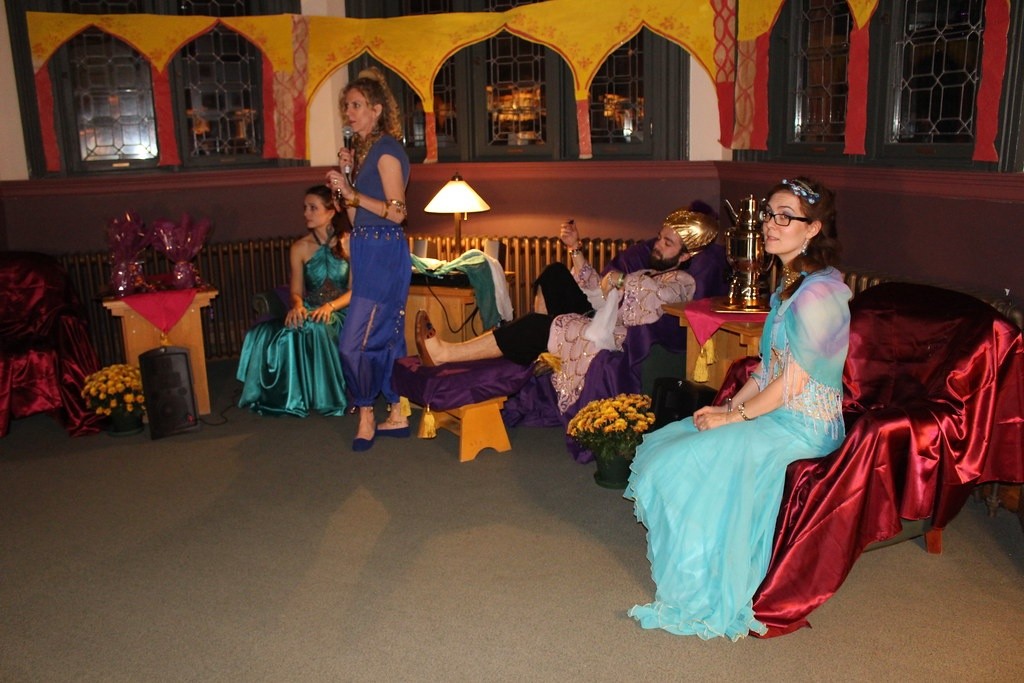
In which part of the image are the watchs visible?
[737,401,752,422]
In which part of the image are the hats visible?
[662,210,719,257]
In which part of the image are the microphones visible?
[339,124,352,175]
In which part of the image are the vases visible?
[101,410,144,436]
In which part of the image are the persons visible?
[231,184,354,419]
[416,206,717,378]
[624,173,854,648]
[325,77,413,452]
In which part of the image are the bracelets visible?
[725,396,735,413]
[348,188,363,208]
[326,300,337,312]
[568,247,583,258]
[608,269,625,289]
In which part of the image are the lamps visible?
[425,173,489,271]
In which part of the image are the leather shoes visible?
[414,309,437,368]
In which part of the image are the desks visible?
[662,296,772,400]
[404,272,510,359]
[101,285,221,417]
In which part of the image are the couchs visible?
[0,252,89,424]
[713,283,1012,580]
[392,236,731,432]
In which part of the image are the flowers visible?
[81,364,149,424]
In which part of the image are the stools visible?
[412,396,513,462]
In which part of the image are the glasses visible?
[758,209,810,227]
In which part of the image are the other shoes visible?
[375,425,410,438]
[352,421,377,452]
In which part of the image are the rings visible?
[699,424,703,430]
[333,178,338,186]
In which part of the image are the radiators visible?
[52,235,297,367]
[404,234,637,317]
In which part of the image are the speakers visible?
[139,341,199,442]
